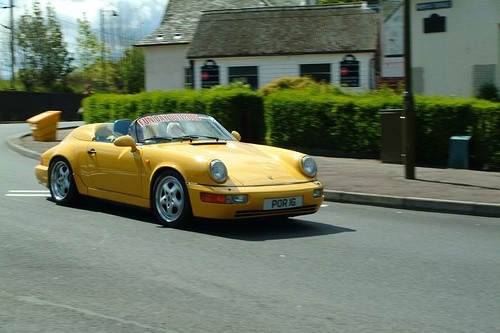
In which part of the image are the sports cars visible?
[35,113,324,227]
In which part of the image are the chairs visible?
[95,122,168,142]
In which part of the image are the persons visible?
[166,122,184,137]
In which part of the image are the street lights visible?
[99,8,120,89]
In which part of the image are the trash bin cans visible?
[448,136,472,170]
[376,108,405,163]
[27,111,62,141]
